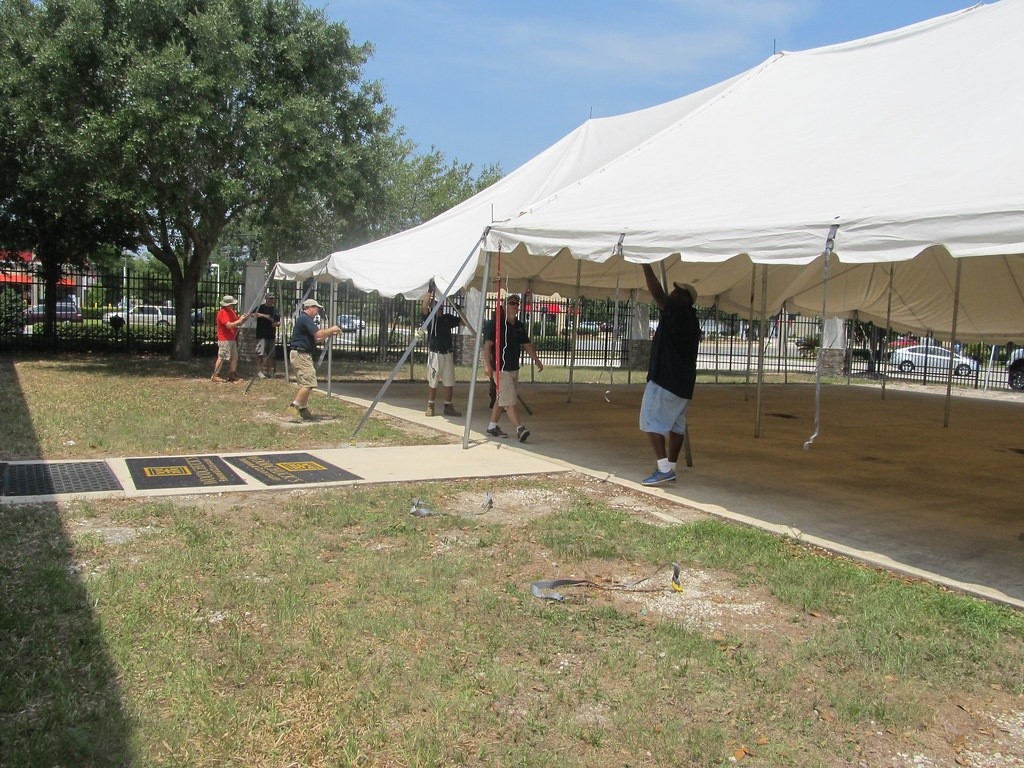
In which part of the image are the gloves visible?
[428,279,435,293]
[454,303,461,311]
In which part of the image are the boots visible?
[444,403,462,417]
[425,403,434,416]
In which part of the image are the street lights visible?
[211,263,220,297]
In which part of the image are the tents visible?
[221,0,1024,443]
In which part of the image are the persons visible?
[289,299,342,420]
[251,293,281,378]
[420,279,466,416]
[638,262,700,486]
[484,295,543,442]
[212,296,247,382]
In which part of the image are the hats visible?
[303,298,323,308]
[503,294,520,304]
[265,293,274,299]
[220,295,238,306]
[673,281,698,305]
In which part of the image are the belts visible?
[430,349,453,354]
[290,347,297,350]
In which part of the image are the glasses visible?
[505,301,519,306]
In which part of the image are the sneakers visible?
[643,467,675,485]
[517,426,530,442]
[486,425,508,438]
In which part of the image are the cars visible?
[890,346,977,377]
[341,315,365,329]
[336,316,357,333]
[1005,349,1024,391]
[103,306,176,326]
[22,302,84,322]
[568,321,626,332]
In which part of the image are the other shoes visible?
[299,407,317,420]
[228,371,238,381]
[258,371,266,379]
[211,373,226,383]
[267,371,273,378]
[285,405,303,420]
[489,403,494,410]
